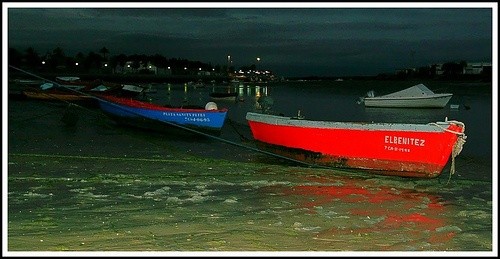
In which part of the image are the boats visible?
[24,82,152,104]
[246,111,464,178]
[352,84,453,109]
[210,92,237,101]
[89,90,229,134]
